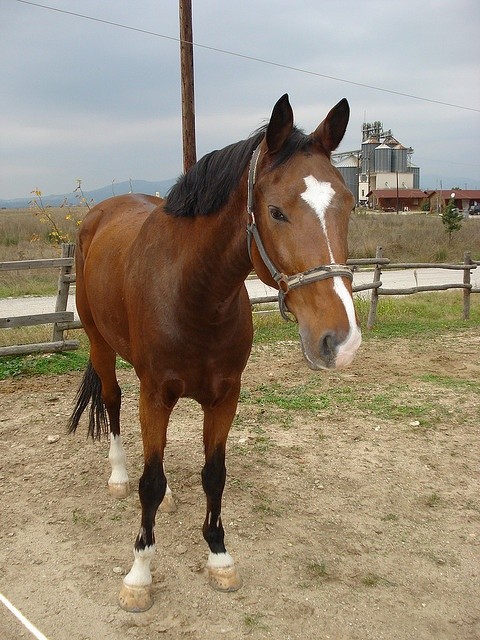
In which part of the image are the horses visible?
[66,91,363,614]
